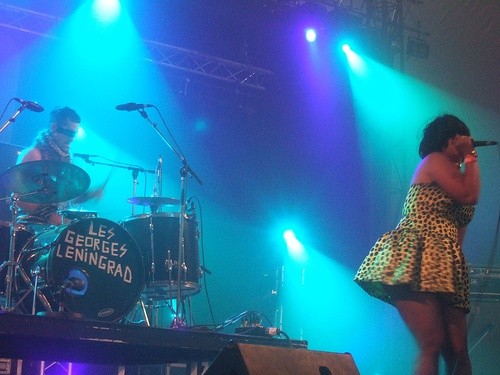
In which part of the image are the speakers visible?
[440,298,500,375]
[203,338,361,375]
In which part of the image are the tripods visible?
[127,167,178,328]
[0,191,52,314]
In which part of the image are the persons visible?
[354,115,479,375]
[13,107,102,227]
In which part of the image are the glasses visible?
[57,127,77,138]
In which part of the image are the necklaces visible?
[52,144,69,157]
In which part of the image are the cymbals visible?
[0,160,91,203]
[127,196,180,205]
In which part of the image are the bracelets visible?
[464,157,476,163]
[472,151,477,157]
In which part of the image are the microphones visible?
[72,153,95,158]
[16,97,44,112]
[235,327,280,337]
[472,140,496,146]
[116,103,152,111]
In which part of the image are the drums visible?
[15,218,145,321]
[121,213,200,299]
[60,209,97,220]
[0,221,57,263]
